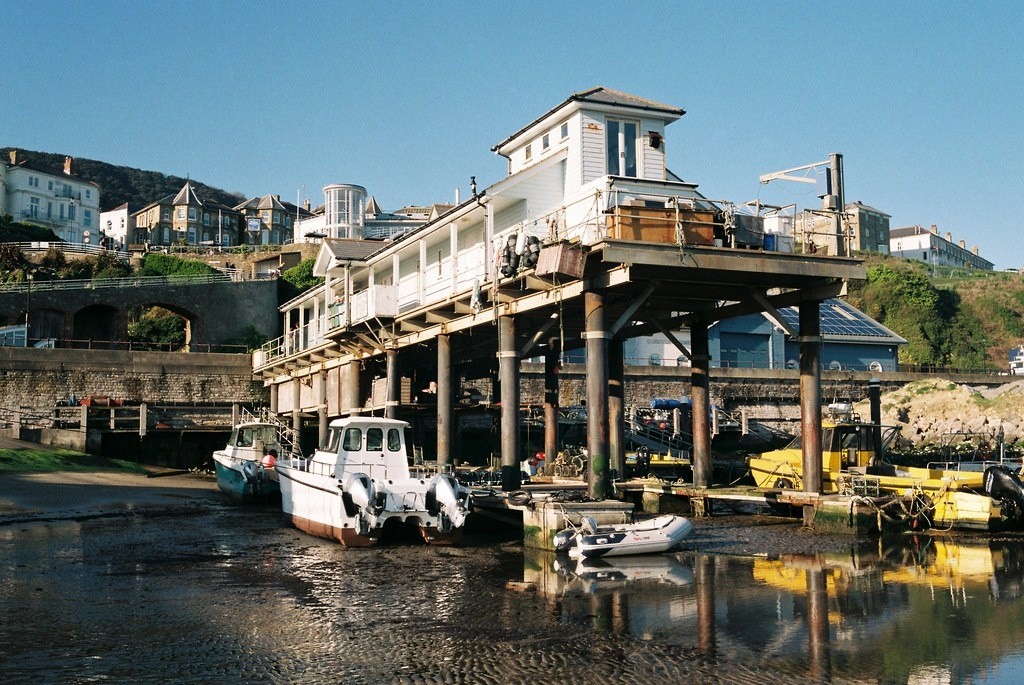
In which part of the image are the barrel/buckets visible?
[763,233,794,253]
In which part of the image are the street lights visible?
[69,195,75,245]
[26,270,33,346]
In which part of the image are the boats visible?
[414,391,777,486]
[754,536,1024,625]
[553,514,692,558]
[213,422,296,506]
[273,382,473,547]
[745,370,1024,531]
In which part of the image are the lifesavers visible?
[772,477,795,489]
[508,489,533,507]
[868,456,879,467]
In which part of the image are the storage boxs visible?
[763,215,795,252]
